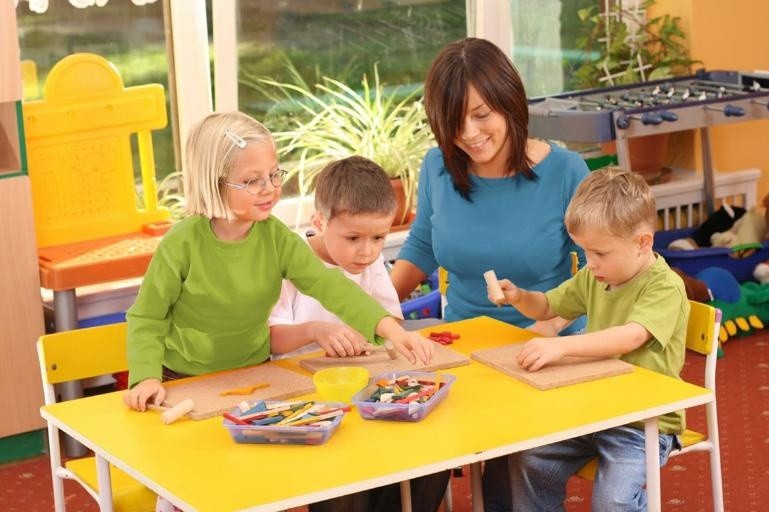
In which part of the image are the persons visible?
[481,165,693,512]
[267,155,411,511]
[123,107,433,511]
[395,33,596,510]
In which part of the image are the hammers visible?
[145,399,195,425]
[483,270,505,308]
[361,339,398,360]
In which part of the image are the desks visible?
[39,316,716,512]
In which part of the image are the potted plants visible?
[563,0,706,183]
[238,48,445,227]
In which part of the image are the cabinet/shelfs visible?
[1,0,48,465]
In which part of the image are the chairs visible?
[436,253,578,324]
[34,321,180,512]
[573,300,726,512]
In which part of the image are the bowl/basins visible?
[314,366,366,403]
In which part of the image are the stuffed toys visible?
[651,196,769,359]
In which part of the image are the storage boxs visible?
[654,228,769,284]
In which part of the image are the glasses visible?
[223,168,289,194]
